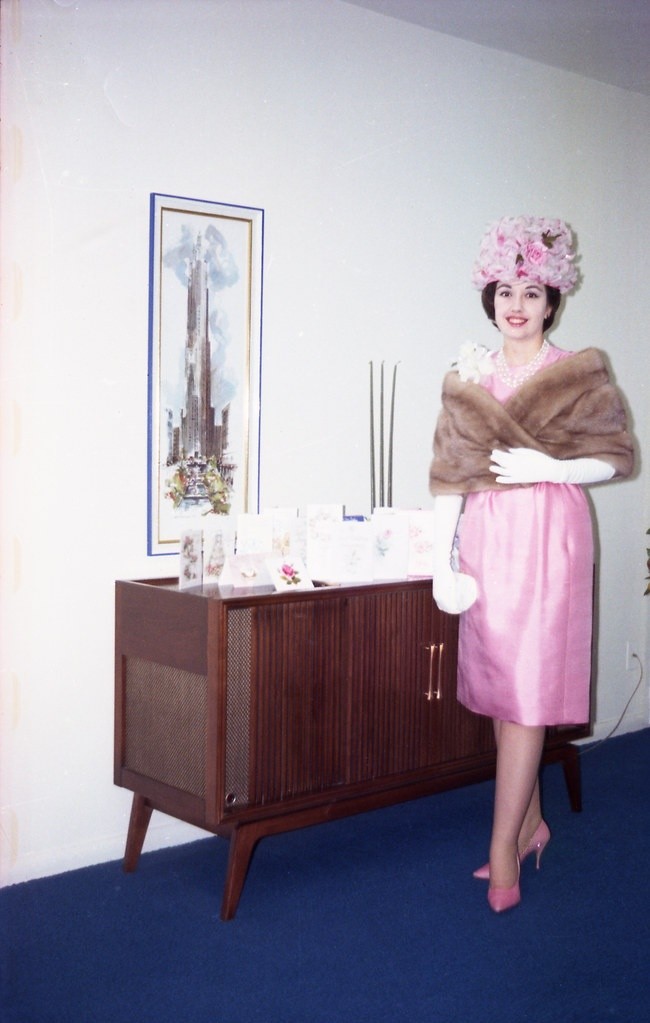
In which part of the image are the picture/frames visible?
[147,192,265,560]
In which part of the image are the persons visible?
[427,210,635,912]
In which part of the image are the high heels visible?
[487,851,520,913]
[473,819,551,879]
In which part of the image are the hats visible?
[473,217,577,293]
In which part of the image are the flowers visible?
[448,340,496,385]
[470,215,581,294]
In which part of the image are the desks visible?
[113,576,590,920]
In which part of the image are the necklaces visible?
[496,340,548,388]
